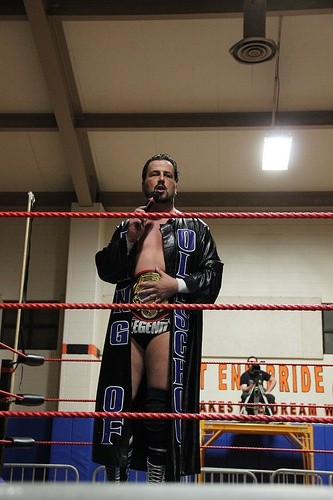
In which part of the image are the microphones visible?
[135,192,159,228]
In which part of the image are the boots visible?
[146,458,169,482]
[104,448,132,483]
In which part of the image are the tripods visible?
[240,378,273,416]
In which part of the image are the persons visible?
[92,154,223,483]
[239,356,275,415]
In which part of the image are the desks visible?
[200,420,315,485]
